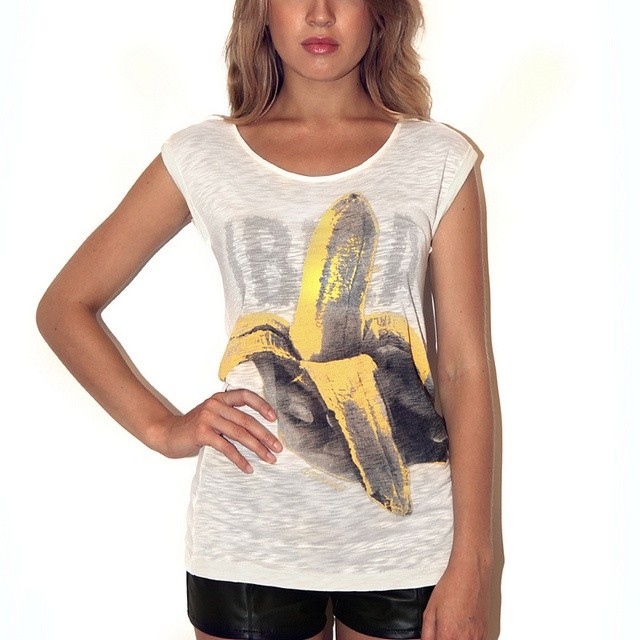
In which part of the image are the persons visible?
[32,0,495,640]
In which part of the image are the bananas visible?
[219,194,435,518]
